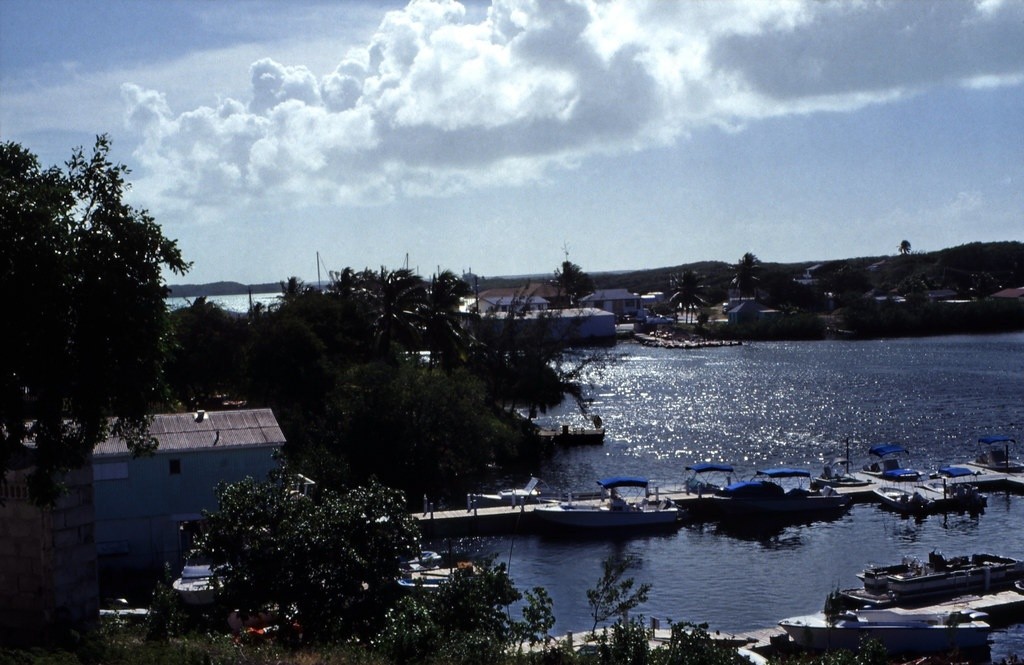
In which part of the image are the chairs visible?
[467,478,539,511]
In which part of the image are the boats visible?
[855,546,1024,596]
[403,548,442,572]
[225,604,304,646]
[664,434,1024,516]
[834,584,898,609]
[393,560,486,597]
[100,597,158,625]
[776,582,996,653]
[173,551,234,604]
[532,476,690,524]
[471,474,599,508]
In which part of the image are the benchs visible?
[562,490,610,507]
